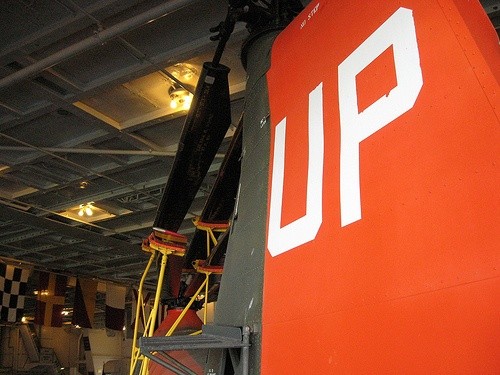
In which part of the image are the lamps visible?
[169,88,192,110]
[78,204,93,216]
